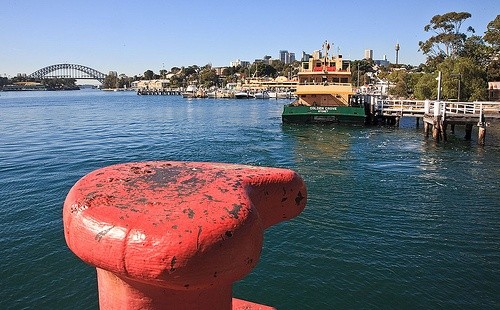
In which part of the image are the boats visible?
[181,81,289,100]
[281,39,367,125]
[2,82,48,91]
